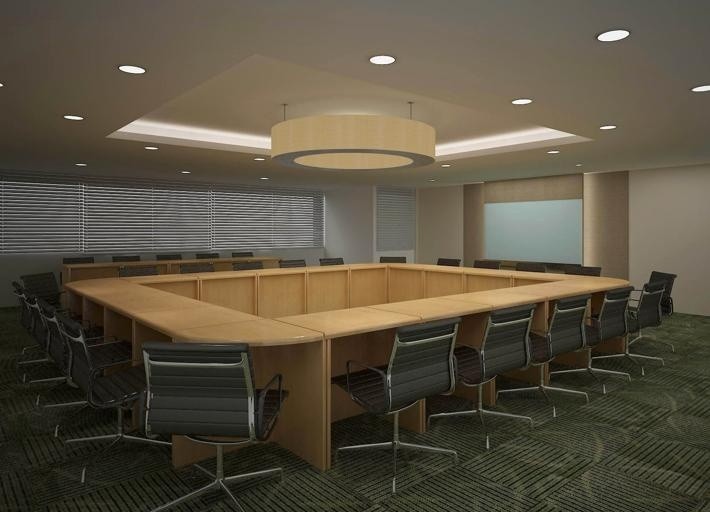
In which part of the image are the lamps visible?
[271,102,436,177]
